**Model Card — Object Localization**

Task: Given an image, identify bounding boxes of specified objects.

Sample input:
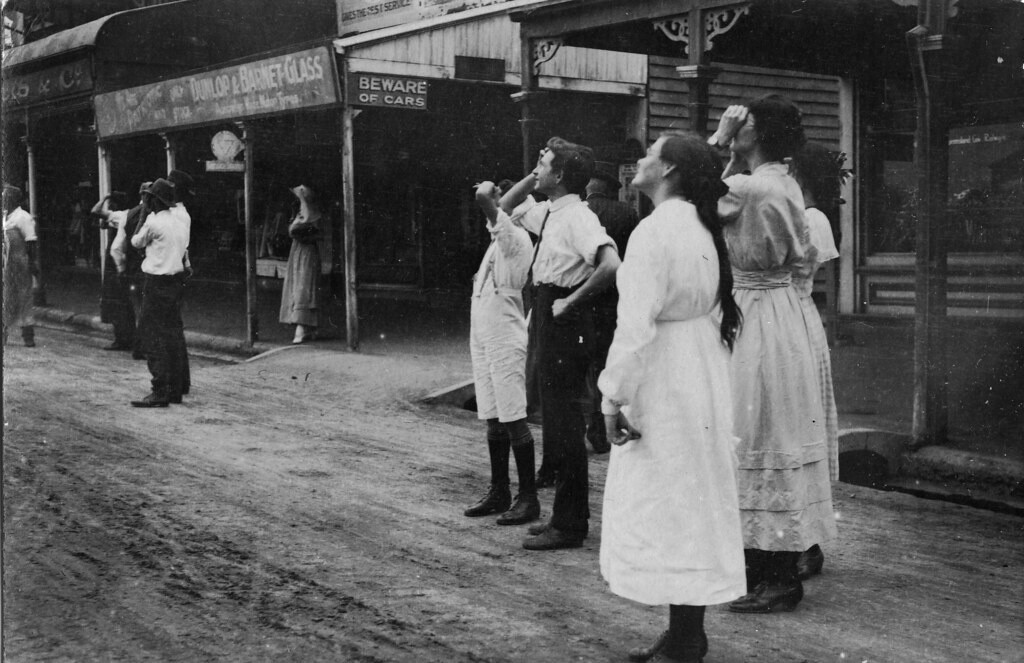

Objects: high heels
[292,329,319,343]
[728,563,802,613]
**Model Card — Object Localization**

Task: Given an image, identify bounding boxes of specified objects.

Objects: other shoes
[22,326,36,347]
[522,517,588,551]
[535,464,559,489]
[629,629,708,663]
[587,412,611,454]
[104,342,130,351]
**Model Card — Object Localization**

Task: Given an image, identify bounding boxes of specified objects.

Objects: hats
[140,178,177,208]
[290,184,315,205]
[167,170,196,196]
[591,161,622,189]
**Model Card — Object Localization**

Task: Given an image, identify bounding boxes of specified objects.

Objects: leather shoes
[168,390,182,403]
[496,489,540,525]
[796,544,824,581]
[464,480,512,516]
[131,392,170,407]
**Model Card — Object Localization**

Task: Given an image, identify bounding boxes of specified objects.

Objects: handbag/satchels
[290,223,324,245]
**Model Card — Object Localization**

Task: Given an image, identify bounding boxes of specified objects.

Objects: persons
[279,181,322,343]
[131,169,194,406]
[597,127,741,663]
[92,182,155,360]
[3,186,37,345]
[463,178,541,522]
[584,92,849,611]
[498,138,621,550]
